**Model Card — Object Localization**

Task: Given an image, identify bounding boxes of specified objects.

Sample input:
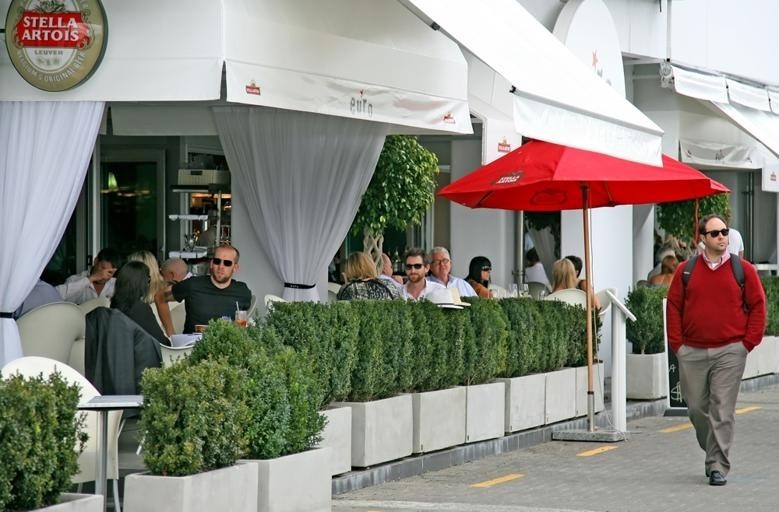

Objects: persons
[646,223,746,289]
[12,244,252,347]
[664,214,768,486]
[329,232,601,311]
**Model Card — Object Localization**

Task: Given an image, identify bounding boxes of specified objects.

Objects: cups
[493,283,547,300]
[235,311,247,328]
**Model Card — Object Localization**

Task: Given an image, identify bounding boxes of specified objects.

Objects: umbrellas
[436,140,733,431]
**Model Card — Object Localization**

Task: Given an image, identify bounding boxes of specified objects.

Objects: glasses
[482,266,492,271]
[406,264,421,269]
[704,229,729,237]
[213,258,232,266]
[433,259,449,264]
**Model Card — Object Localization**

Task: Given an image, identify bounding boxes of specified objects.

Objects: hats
[429,288,464,310]
[450,288,472,306]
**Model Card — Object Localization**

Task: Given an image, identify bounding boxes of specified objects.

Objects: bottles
[386,248,408,273]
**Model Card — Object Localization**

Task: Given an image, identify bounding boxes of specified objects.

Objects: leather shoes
[710,471,726,484]
